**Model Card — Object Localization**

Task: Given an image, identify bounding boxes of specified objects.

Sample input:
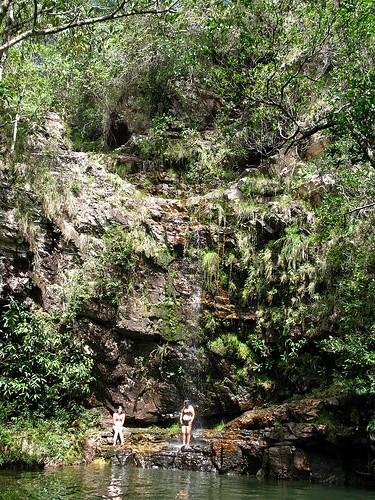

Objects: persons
[112,405,125,446]
[179,400,195,445]
